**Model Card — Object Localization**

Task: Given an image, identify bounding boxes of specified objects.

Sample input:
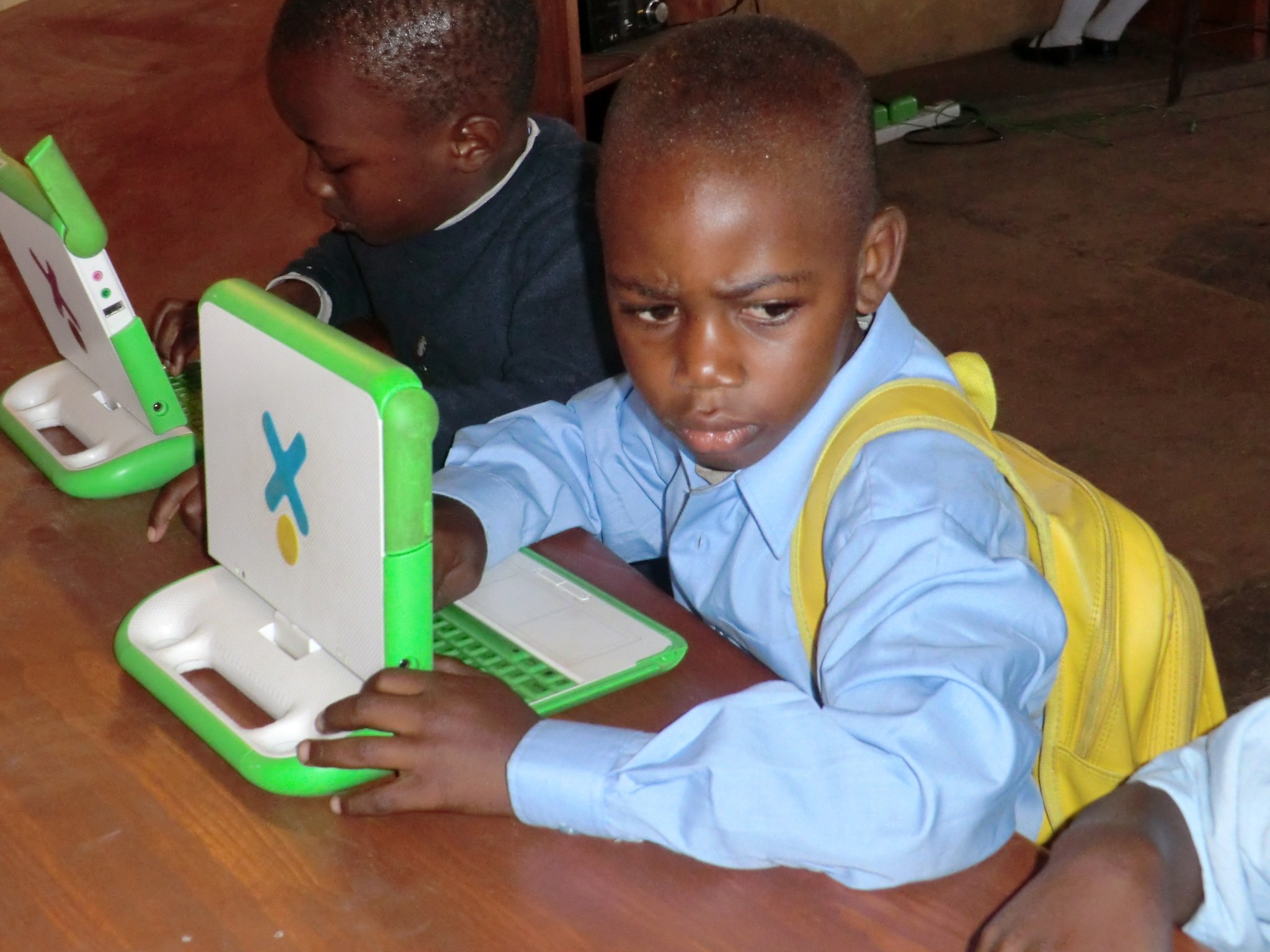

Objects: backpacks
[793,351,1230,852]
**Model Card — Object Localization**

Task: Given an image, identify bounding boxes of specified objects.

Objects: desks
[0,0,1210,952]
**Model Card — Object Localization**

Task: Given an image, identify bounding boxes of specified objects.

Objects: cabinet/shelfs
[567,0,713,150]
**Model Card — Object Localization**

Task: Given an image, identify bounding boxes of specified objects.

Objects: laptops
[0,133,203,499]
[113,277,689,795]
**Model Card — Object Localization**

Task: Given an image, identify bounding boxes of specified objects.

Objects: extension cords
[873,100,963,146]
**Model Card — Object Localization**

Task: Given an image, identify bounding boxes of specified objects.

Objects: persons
[146,0,630,543]
[301,12,1068,891]
[974,694,1270,952]
[1008,0,1147,65]
